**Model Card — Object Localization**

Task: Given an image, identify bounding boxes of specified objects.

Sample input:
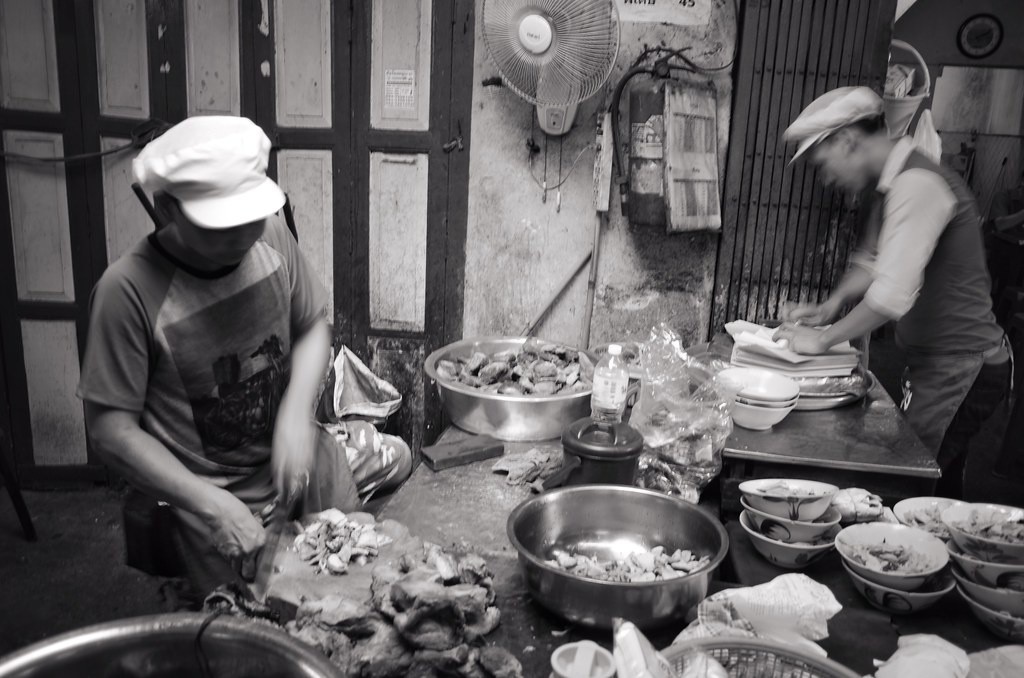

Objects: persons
[75,116,362,601]
[771,87,1015,500]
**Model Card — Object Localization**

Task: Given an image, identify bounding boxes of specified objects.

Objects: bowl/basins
[0,610,350,678]
[423,336,594,442]
[738,478,1024,642]
[507,482,729,628]
[715,367,800,430]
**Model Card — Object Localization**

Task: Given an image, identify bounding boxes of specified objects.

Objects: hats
[131,115,286,230]
[783,86,885,168]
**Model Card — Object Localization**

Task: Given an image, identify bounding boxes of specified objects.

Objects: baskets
[659,636,862,678]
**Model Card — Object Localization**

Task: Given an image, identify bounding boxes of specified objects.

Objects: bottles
[589,344,630,425]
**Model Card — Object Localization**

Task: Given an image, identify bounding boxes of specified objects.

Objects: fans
[479,0,622,135]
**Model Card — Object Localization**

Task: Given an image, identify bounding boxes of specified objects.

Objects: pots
[540,418,644,490]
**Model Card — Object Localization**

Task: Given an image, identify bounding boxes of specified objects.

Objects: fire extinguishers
[611,46,695,228]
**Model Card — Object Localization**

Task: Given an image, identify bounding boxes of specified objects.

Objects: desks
[724,368,938,506]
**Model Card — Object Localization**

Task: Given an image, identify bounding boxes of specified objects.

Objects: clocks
[956,14,1002,58]
